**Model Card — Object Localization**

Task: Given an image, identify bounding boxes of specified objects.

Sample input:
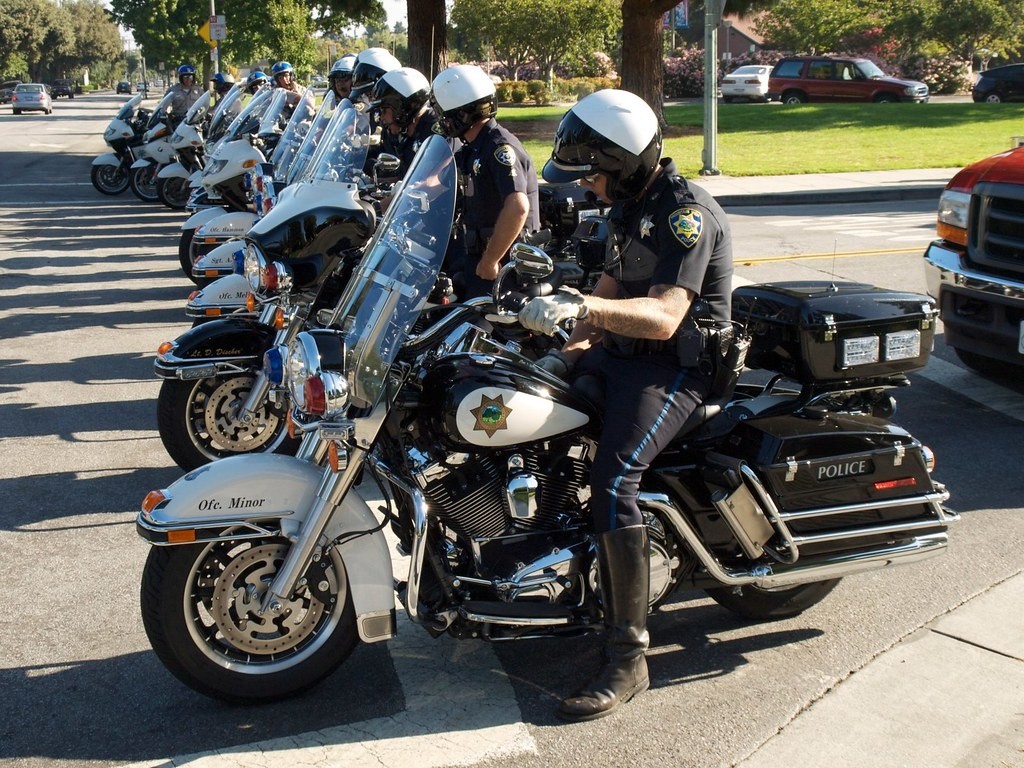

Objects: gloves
[534,348,573,378]
[518,285,589,338]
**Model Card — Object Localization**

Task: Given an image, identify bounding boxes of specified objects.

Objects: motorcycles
[87,77,961,703]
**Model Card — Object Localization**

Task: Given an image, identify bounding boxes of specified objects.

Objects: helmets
[430,64,498,137]
[178,64,196,84]
[542,89,663,201]
[328,48,430,129]
[272,61,292,79]
[245,71,268,91]
[209,72,234,94]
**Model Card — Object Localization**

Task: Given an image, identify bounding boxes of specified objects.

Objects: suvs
[768,56,930,104]
[0,80,23,104]
[51,79,74,99]
[921,142,1024,367]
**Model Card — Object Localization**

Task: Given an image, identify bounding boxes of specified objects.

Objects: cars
[156,80,163,87]
[137,82,150,92]
[972,63,1024,103]
[117,82,131,95]
[12,84,53,115]
[721,65,775,103]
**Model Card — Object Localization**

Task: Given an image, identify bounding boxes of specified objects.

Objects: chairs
[842,66,852,81]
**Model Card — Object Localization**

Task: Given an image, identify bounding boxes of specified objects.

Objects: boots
[553,524,650,722]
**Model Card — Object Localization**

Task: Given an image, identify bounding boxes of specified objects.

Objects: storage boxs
[571,216,609,273]
[729,281,943,382]
[537,182,612,228]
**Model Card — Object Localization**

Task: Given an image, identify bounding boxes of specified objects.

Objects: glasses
[331,74,352,82]
[584,173,600,188]
[181,74,194,79]
[373,108,386,119]
[248,80,265,90]
[277,74,290,78]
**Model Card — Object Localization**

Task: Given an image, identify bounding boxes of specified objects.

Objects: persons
[160,47,538,303]
[524,88,733,721]
